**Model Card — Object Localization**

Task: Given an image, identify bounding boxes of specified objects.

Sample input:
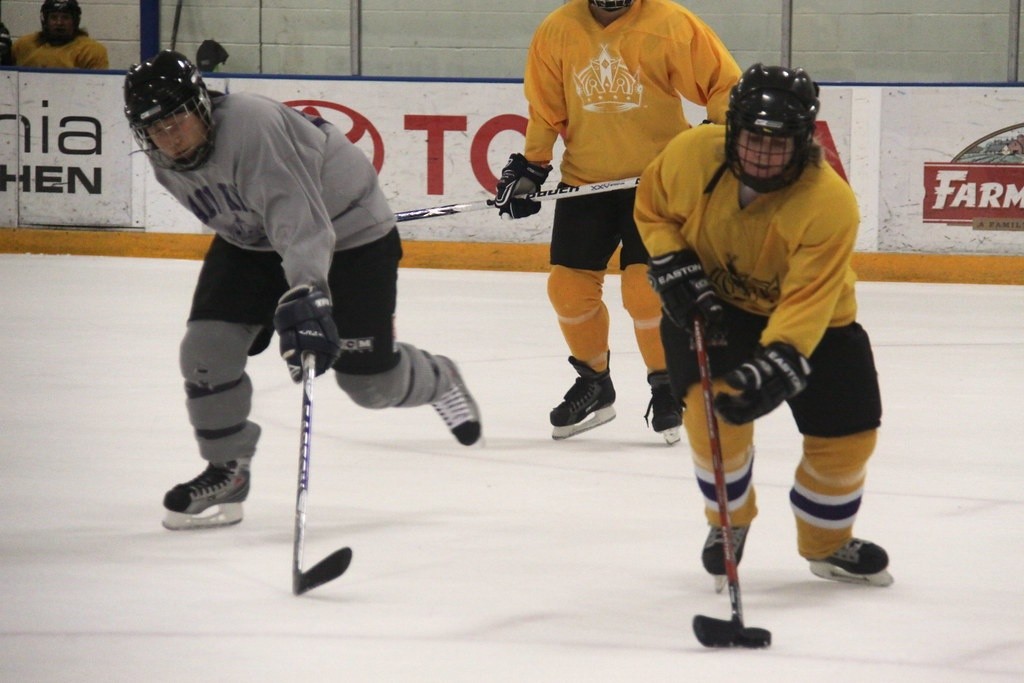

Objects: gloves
[646,248,727,341]
[495,153,553,221]
[713,341,816,424]
[273,285,340,384]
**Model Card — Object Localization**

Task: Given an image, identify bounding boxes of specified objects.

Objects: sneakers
[809,538,893,587]
[432,355,487,451]
[550,350,617,440]
[162,451,251,530]
[702,525,752,594]
[645,369,690,444]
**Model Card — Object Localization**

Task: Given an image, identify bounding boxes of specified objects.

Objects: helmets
[587,0,632,12]
[40,0,81,44]
[724,62,820,193]
[123,50,216,173]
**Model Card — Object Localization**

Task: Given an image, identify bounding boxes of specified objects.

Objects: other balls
[736,628,772,648]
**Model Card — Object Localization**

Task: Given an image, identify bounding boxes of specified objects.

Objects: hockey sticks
[691,308,744,649]
[291,351,353,595]
[394,176,640,222]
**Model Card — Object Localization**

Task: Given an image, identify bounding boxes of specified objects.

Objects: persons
[496,0,742,445]
[12,0,110,70]
[123,50,483,532]
[633,63,895,593]
